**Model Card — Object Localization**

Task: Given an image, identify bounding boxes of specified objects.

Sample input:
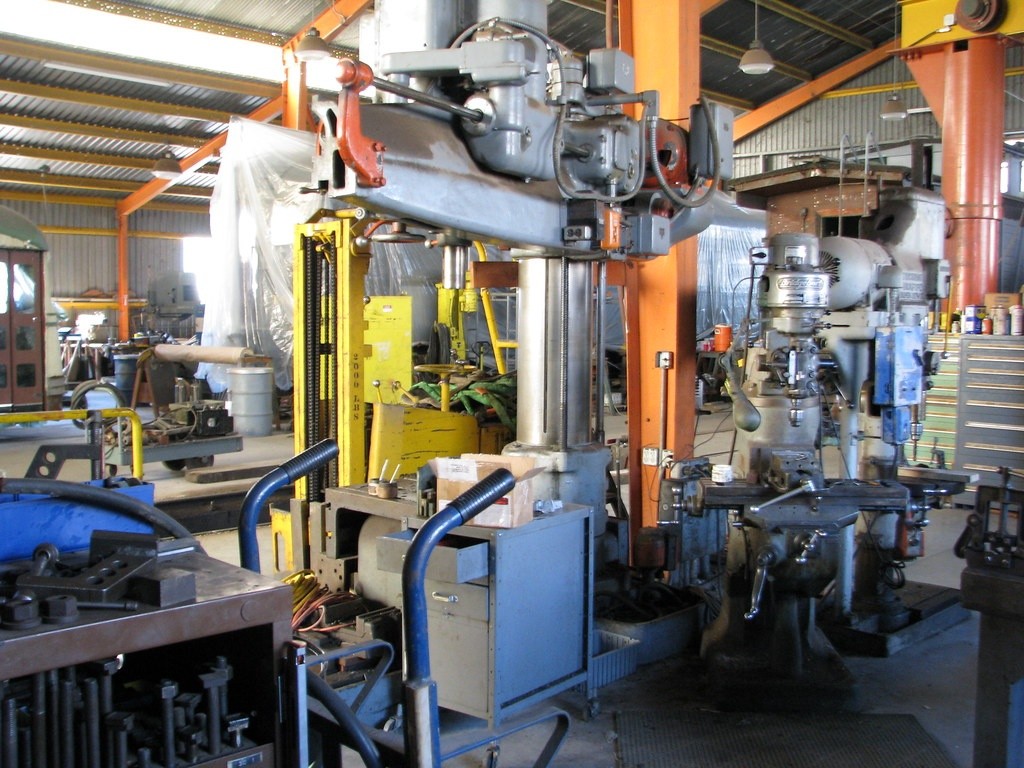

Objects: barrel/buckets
[226,366,274,436]
[112,355,141,407]
[714,324,732,353]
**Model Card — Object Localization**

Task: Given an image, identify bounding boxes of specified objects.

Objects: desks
[695,350,725,403]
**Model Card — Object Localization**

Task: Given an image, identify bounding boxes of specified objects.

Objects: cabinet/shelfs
[375,502,597,726]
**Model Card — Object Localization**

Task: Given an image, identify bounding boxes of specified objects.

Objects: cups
[711,464,732,482]
[368,478,397,498]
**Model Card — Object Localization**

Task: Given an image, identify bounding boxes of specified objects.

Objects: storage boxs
[985,293,1022,314]
[427,452,547,529]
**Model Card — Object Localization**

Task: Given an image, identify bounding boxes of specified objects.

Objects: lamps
[878,1,909,123]
[738,0,776,76]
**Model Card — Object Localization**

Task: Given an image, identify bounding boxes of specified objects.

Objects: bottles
[704,340,708,351]
[1011,305,1023,335]
[951,308,961,333]
[710,338,714,351]
